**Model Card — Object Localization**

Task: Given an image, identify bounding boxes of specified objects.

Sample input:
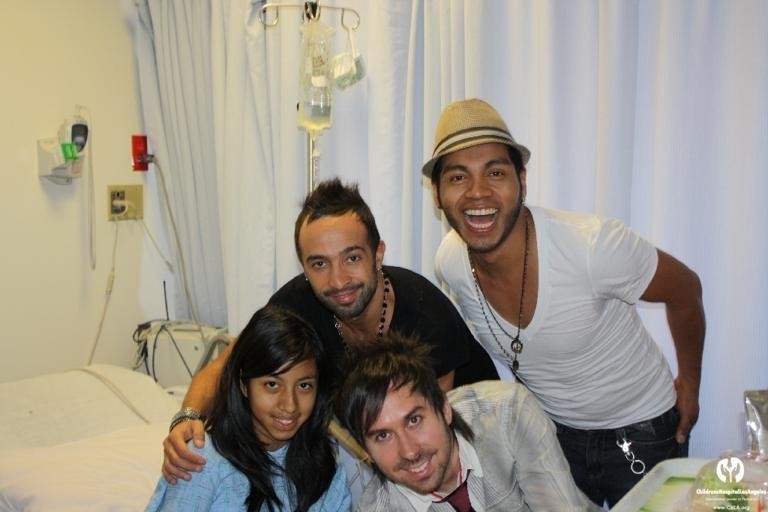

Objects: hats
[418,96,535,179]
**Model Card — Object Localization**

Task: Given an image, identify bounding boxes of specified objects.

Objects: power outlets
[130,135,149,171]
[106,184,144,222]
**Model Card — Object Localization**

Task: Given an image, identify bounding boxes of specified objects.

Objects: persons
[133,305,366,512]
[418,97,706,512]
[160,174,506,484]
[317,322,596,512]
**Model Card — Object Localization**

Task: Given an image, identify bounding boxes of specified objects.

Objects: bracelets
[166,403,205,437]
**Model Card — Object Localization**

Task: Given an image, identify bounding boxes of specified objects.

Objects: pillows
[0,422,177,512]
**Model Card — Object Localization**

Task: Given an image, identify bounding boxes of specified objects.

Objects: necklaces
[465,248,524,372]
[465,210,533,353]
[320,271,389,353]
